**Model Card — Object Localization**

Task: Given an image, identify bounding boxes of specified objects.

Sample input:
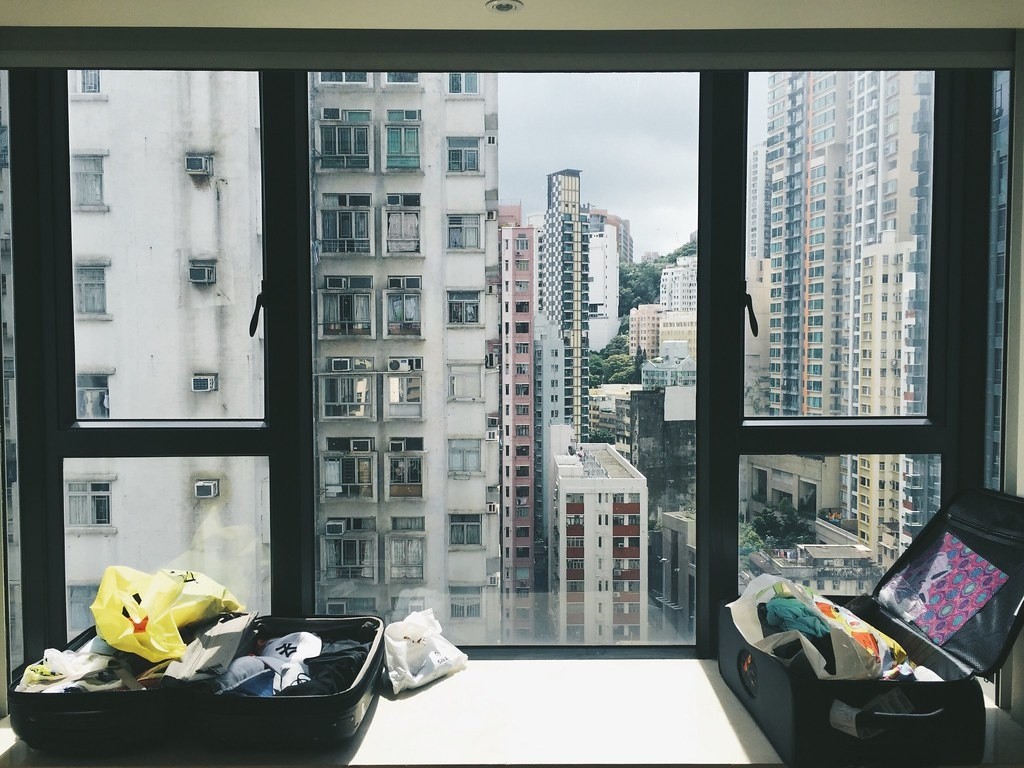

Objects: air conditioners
[890,359,897,365]
[488,416,500,427]
[614,569,621,576]
[519,580,526,585]
[516,497,527,505]
[326,277,348,290]
[194,479,219,499]
[486,573,498,586]
[326,601,346,615]
[350,440,372,453]
[326,520,346,536]
[386,195,402,207]
[191,376,215,392]
[408,601,424,614]
[618,543,625,548]
[387,358,409,372]
[320,107,342,120]
[331,357,352,371]
[488,353,496,367]
[486,502,497,513]
[486,135,496,147]
[613,592,620,598]
[488,284,497,294]
[389,440,405,453]
[188,267,213,282]
[404,109,420,122]
[485,428,497,441]
[405,277,422,289]
[185,156,209,173]
[486,211,496,221]
